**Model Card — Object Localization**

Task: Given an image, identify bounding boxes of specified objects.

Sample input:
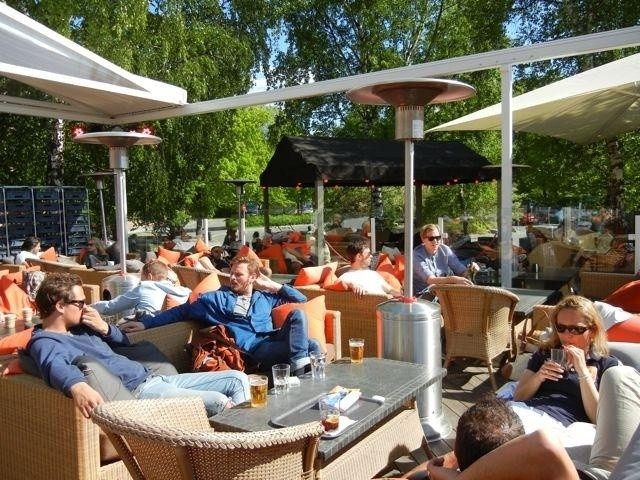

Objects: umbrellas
[424,52,640,145]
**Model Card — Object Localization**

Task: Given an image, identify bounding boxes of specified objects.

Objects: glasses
[425,236,441,241]
[556,322,594,335]
[63,299,84,310]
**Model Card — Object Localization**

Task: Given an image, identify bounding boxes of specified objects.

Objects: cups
[22,306,33,323]
[348,337,365,364]
[309,350,327,380]
[272,363,290,395]
[319,395,340,429]
[550,348,569,379]
[249,375,269,408]
[4,314,16,329]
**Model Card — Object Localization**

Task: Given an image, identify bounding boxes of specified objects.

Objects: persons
[14,208,640,480]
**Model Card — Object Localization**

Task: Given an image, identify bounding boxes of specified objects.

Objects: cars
[246,201,260,213]
[516,208,601,225]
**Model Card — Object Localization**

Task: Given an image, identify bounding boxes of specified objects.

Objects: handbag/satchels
[191,324,246,372]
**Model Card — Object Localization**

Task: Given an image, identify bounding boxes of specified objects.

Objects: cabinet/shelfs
[7,187,91,253]
